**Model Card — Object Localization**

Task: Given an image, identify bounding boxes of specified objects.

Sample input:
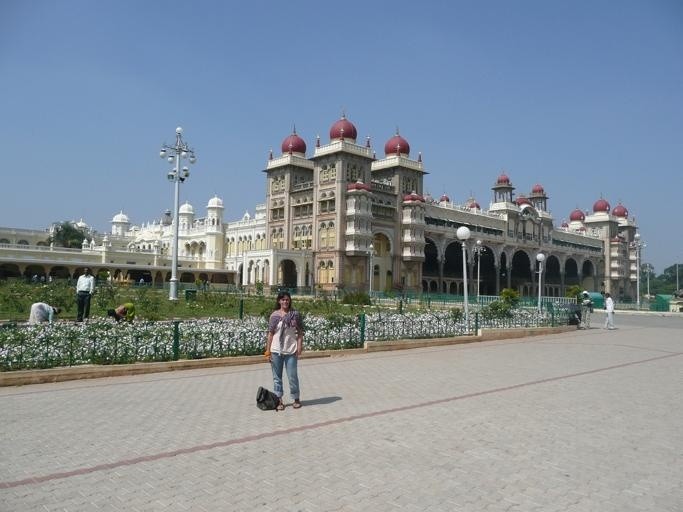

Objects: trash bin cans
[186,291,197,305]
[583,292,604,308]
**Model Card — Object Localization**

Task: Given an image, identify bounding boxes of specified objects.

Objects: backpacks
[257,386,279,410]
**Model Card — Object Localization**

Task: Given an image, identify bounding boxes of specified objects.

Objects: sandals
[293,402,301,408]
[276,403,284,410]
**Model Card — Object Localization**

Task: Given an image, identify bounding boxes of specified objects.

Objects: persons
[73,268,97,325]
[139,277,144,287]
[30,273,53,285]
[576,290,594,330]
[262,291,304,411]
[602,292,617,329]
[108,302,135,324]
[27,300,61,323]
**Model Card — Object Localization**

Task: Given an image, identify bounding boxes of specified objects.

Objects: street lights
[537,253,545,314]
[456,226,470,335]
[631,232,651,305]
[159,127,196,300]
[365,244,377,299]
